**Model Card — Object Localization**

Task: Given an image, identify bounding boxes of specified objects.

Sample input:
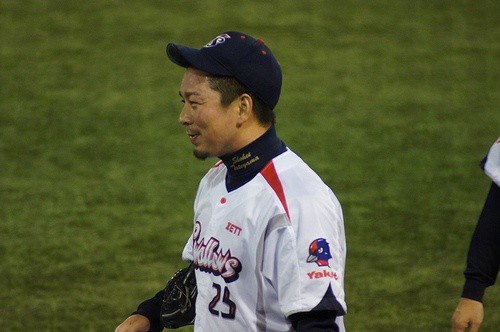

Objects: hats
[166,31,282,111]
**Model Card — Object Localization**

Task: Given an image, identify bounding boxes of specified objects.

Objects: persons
[450,137,500,332]
[114,32,348,332]
